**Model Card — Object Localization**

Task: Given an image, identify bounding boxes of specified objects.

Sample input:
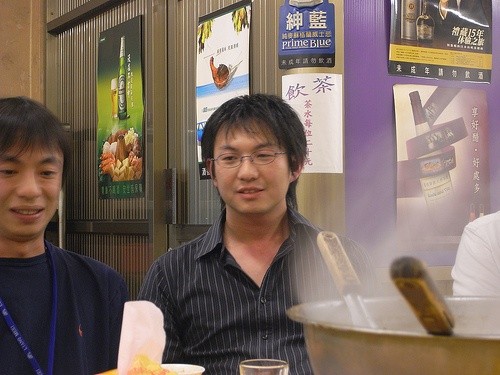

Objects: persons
[136,93,381,375]
[450,211,500,297]
[0,96,132,375]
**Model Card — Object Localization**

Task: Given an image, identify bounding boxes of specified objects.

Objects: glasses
[209,149,288,168]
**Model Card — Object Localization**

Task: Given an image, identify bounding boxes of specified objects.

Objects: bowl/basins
[287,296,500,375]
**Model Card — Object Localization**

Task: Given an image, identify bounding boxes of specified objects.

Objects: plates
[162,364,205,375]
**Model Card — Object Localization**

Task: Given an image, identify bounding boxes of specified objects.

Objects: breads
[127,352,181,375]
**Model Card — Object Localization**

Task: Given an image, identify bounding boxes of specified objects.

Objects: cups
[111,78,118,119]
[239,359,289,375]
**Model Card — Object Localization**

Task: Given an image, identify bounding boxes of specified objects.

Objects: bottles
[415,0,435,43]
[118,36,128,120]
[410,91,455,216]
